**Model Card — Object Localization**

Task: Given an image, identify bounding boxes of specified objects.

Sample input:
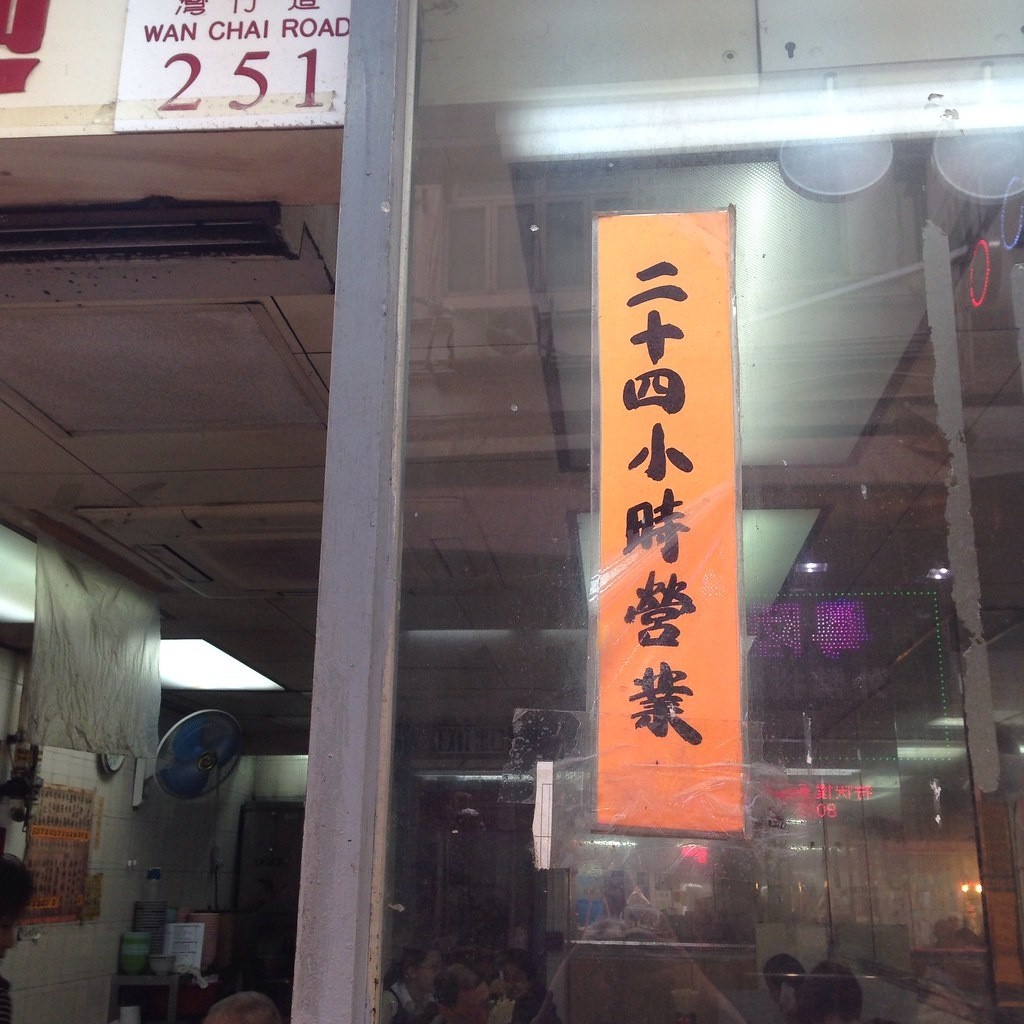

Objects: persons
[378,884,1003,1023]
[1,852,36,1024]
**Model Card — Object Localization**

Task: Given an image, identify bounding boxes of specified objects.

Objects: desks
[106,961,253,1024]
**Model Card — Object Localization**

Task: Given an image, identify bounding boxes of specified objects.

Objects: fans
[131,708,246,809]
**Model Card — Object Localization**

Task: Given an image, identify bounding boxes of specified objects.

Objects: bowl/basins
[133,899,168,975]
[149,955,175,976]
[176,906,193,923]
[119,931,151,976]
[190,911,219,972]
